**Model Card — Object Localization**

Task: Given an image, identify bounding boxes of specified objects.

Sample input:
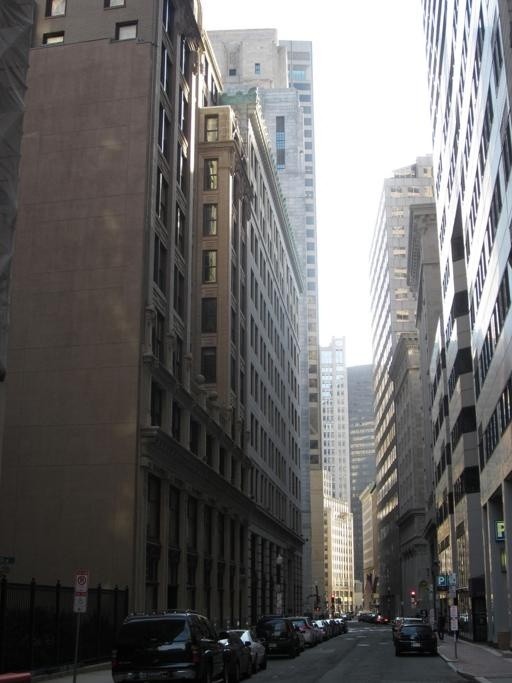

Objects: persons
[437,611,445,640]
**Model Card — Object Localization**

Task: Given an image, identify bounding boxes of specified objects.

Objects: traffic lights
[414,600,420,606]
[317,595,321,604]
[411,592,416,598]
[331,594,335,603]
[316,606,321,611]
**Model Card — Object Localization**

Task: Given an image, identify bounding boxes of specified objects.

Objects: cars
[392,615,439,659]
[357,611,390,624]
[108,608,354,682]
